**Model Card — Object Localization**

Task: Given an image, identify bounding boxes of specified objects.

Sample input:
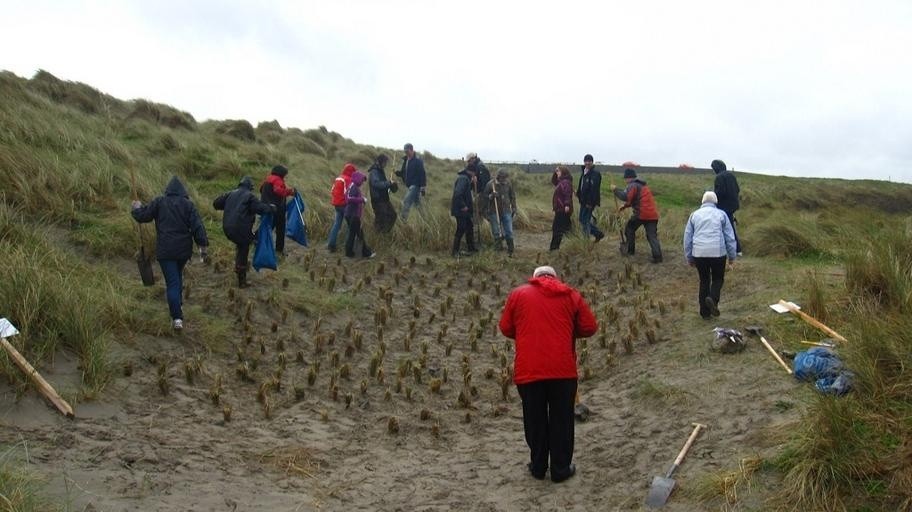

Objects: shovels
[473,175,482,251]
[768,299,849,345]
[744,324,794,376]
[609,171,629,257]
[128,169,156,287]
[644,422,708,511]
[0,316,75,421]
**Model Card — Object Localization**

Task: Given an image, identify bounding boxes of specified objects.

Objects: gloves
[510,208,517,218]
[362,196,368,208]
[131,199,142,210]
[420,187,426,197]
[288,187,298,197]
[389,181,398,194]
[197,245,211,262]
[489,192,500,200]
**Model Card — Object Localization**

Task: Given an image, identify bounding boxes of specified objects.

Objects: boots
[237,270,252,289]
[495,238,503,251]
[506,238,515,258]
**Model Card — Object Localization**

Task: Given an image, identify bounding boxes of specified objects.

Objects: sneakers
[451,246,479,257]
[345,250,356,258]
[550,463,576,484]
[622,247,636,256]
[328,244,337,252]
[362,252,377,260]
[647,255,663,263]
[528,461,548,481]
[698,294,720,321]
[736,250,743,257]
[172,318,184,330]
[594,231,605,243]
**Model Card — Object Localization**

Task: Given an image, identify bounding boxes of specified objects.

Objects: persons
[259,165,298,257]
[499,264,598,481]
[367,154,397,232]
[450,151,668,265]
[326,162,356,253]
[710,160,744,257]
[395,143,430,225]
[345,171,379,258]
[131,175,211,330]
[214,174,276,288]
[684,191,736,320]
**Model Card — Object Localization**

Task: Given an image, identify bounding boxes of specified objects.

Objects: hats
[583,154,594,162]
[533,266,557,279]
[623,168,638,178]
[465,152,476,162]
